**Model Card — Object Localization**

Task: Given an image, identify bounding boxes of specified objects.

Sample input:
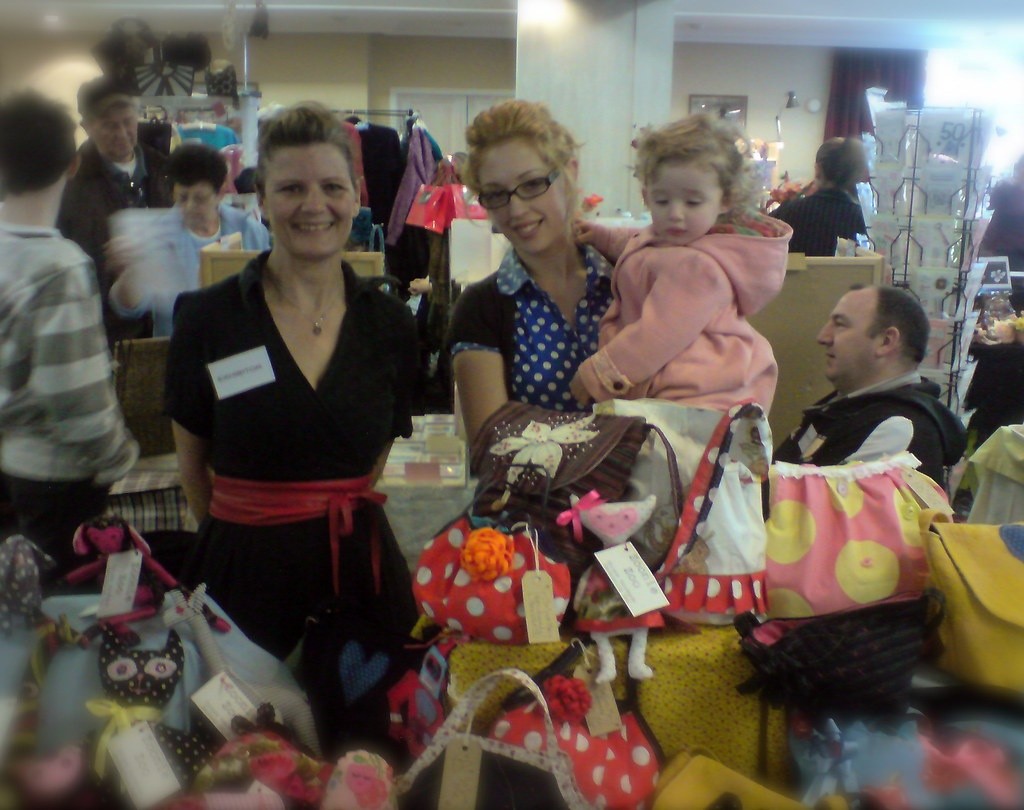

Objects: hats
[78,76,138,123]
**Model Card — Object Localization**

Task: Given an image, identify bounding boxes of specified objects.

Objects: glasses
[478,158,570,209]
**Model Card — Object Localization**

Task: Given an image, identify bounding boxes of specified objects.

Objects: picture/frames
[689,93,750,130]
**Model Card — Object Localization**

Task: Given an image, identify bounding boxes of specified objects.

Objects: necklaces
[263,268,343,337]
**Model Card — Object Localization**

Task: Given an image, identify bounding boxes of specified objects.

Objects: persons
[0,75,1024,775]
[567,112,795,417]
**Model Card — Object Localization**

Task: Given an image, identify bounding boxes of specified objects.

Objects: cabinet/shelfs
[858,104,987,483]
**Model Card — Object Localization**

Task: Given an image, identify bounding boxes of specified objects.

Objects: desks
[112,437,478,566]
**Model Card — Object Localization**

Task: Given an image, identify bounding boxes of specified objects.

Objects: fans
[91,15,160,82]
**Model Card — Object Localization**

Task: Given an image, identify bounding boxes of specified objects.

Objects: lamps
[776,90,801,143]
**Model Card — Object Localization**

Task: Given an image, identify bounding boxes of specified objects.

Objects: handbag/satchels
[137,116,172,154]
[444,160,489,229]
[390,419,1024,810]
[404,161,449,234]
